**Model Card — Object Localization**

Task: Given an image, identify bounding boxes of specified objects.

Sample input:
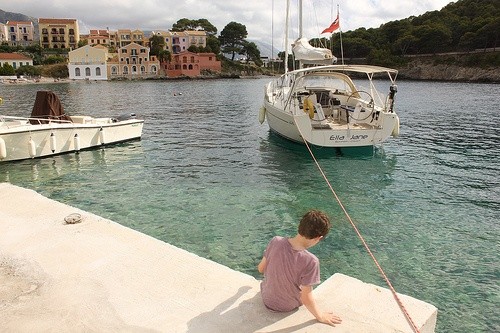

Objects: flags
[322,13,340,34]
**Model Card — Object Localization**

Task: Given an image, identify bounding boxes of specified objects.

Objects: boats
[0,91,145,162]
[259,1,400,148]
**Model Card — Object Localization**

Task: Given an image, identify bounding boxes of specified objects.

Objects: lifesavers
[303,98,314,119]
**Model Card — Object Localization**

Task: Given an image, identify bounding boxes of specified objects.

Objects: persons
[256,209,344,327]
